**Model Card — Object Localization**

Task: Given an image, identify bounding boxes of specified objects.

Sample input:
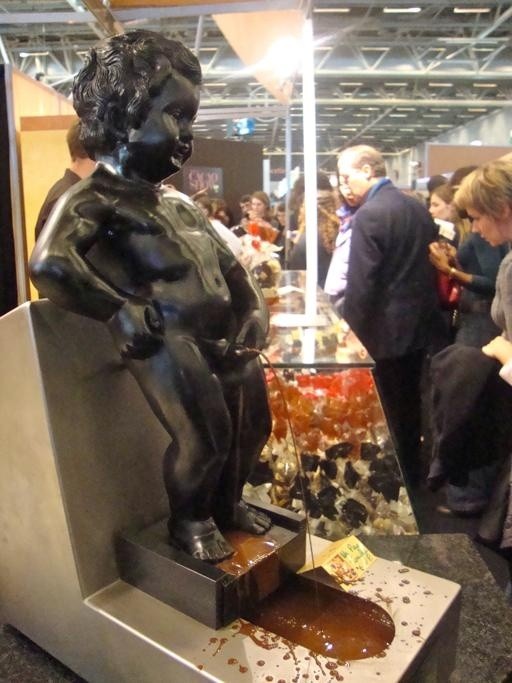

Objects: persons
[28,29,272,563]
[35,118,97,241]
